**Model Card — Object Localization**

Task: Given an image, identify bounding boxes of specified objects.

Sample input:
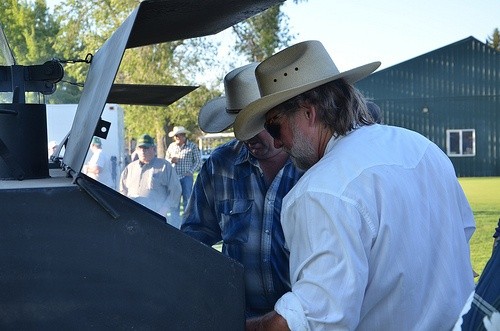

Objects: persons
[451,217,500,331]
[118,132,182,230]
[47,141,60,159]
[81,135,106,181]
[233,40,477,331]
[163,125,202,212]
[179,60,308,319]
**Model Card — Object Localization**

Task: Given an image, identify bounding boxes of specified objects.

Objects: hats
[198,61,261,134]
[137,134,154,149]
[92,136,101,146]
[168,126,191,137]
[234,41,381,142]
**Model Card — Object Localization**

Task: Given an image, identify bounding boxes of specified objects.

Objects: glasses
[264,104,297,140]
